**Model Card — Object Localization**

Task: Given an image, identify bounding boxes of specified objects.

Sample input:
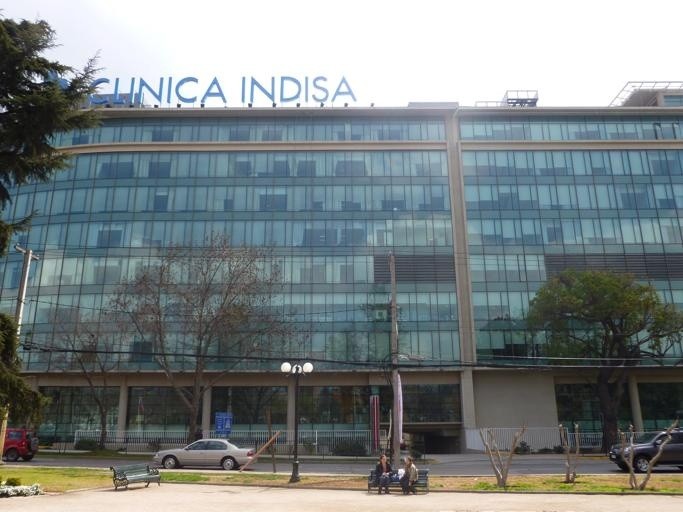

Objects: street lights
[281,361,314,483]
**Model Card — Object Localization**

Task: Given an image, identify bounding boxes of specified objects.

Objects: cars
[153,438,258,470]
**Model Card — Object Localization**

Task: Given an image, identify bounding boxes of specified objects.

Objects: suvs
[608,426,683,473]
[3,427,40,462]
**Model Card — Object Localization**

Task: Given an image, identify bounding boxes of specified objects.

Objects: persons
[399,455,418,495]
[375,453,393,495]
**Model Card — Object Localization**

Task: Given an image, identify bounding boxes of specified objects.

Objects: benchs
[366,469,430,495]
[109,463,161,492]
